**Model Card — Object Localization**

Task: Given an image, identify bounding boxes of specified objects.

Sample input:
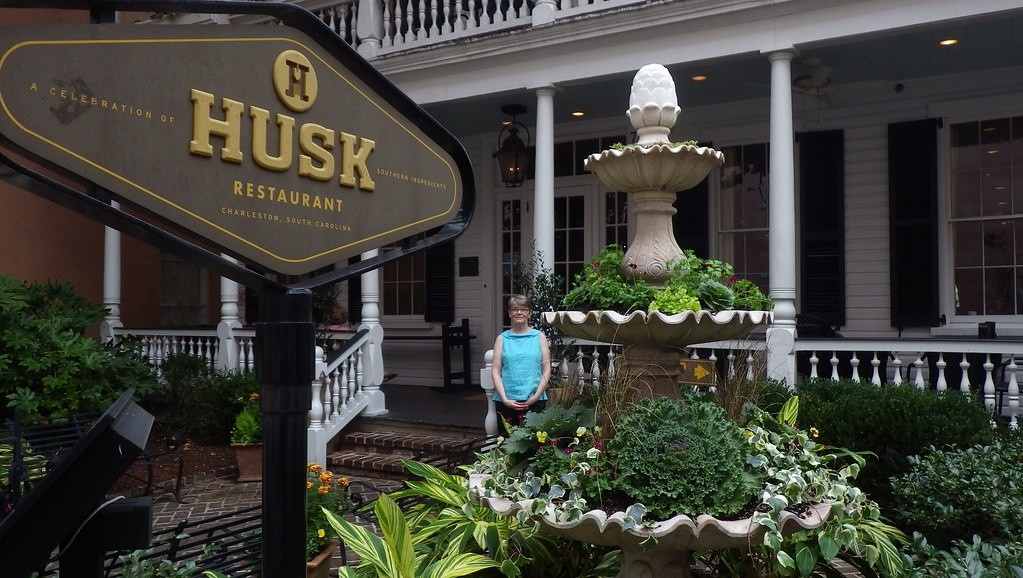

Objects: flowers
[557,242,774,316]
[470,389,879,522]
[305,462,353,564]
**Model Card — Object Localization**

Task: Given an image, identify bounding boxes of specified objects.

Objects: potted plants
[582,138,726,282]
[228,400,266,482]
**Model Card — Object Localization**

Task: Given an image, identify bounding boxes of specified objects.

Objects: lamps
[0,385,156,578]
[491,103,530,188]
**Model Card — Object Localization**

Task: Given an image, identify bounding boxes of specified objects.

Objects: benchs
[0,405,183,507]
[37,504,263,578]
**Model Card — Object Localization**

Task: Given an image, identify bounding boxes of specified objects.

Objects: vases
[456,473,858,578]
[540,307,774,404]
[305,539,340,578]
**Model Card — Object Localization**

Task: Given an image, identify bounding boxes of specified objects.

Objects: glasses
[509,308,530,315]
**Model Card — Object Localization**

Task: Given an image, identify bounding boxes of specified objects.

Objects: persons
[491,294,551,438]
